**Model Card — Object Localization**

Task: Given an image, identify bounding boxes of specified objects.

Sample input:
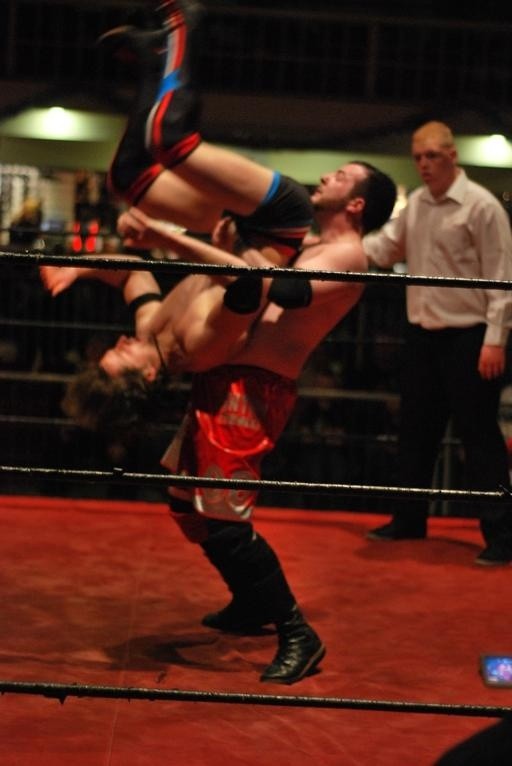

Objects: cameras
[480,654,512,687]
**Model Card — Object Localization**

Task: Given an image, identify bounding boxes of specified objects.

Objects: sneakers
[368,521,426,540]
[473,546,511,565]
[98,23,167,56]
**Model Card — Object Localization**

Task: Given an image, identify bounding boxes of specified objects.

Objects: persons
[362,120,512,564]
[156,157,396,685]
[1,197,512,503]
[62,0,309,405]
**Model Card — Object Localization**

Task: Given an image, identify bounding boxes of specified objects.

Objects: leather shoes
[201,597,263,636]
[257,624,326,682]
